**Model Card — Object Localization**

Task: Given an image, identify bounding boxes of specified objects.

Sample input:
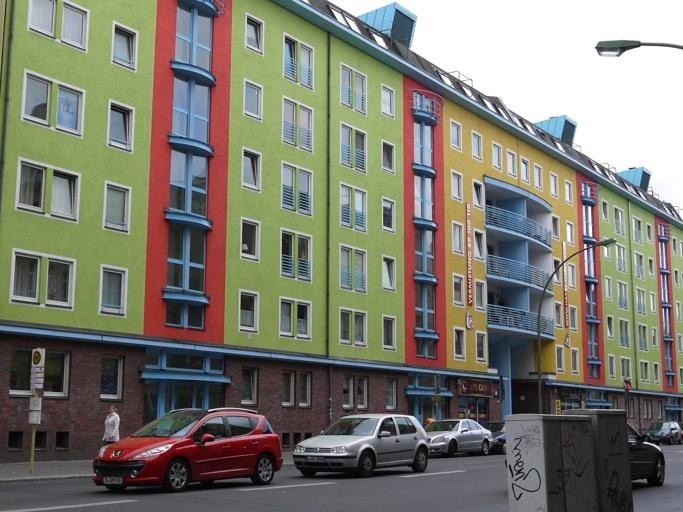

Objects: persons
[95,404,122,477]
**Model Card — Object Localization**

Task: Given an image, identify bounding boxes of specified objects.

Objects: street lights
[595,39,683,59]
[535,238,617,414]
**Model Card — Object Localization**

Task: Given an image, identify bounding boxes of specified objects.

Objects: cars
[641,419,683,445]
[424,418,507,458]
[93,407,283,492]
[292,412,431,478]
[626,421,665,486]
